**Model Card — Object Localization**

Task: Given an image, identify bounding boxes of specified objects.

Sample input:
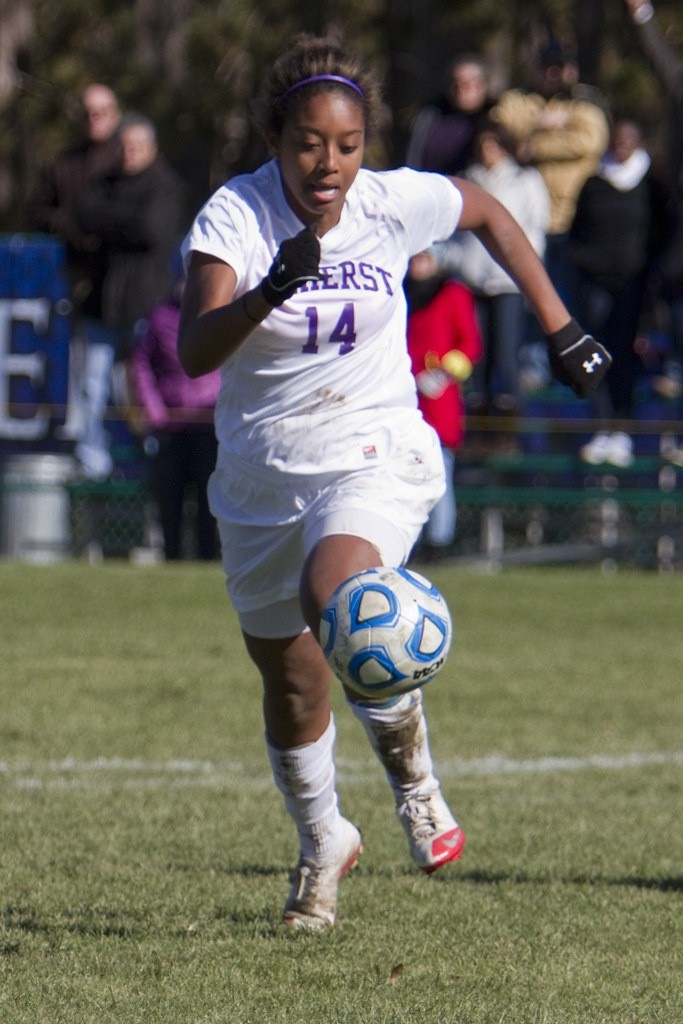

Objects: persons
[399,242,479,558]
[383,54,681,574]
[172,45,611,929]
[128,272,222,563]
[21,71,182,476]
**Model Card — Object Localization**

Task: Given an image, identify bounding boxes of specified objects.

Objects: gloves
[260,222,324,306]
[545,317,613,400]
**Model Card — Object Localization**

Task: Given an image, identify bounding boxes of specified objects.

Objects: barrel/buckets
[0,455,75,562]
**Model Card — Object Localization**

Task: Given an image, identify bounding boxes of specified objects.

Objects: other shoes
[417,545,450,568]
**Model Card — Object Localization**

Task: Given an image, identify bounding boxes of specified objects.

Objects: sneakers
[283,820,364,934]
[395,783,464,874]
